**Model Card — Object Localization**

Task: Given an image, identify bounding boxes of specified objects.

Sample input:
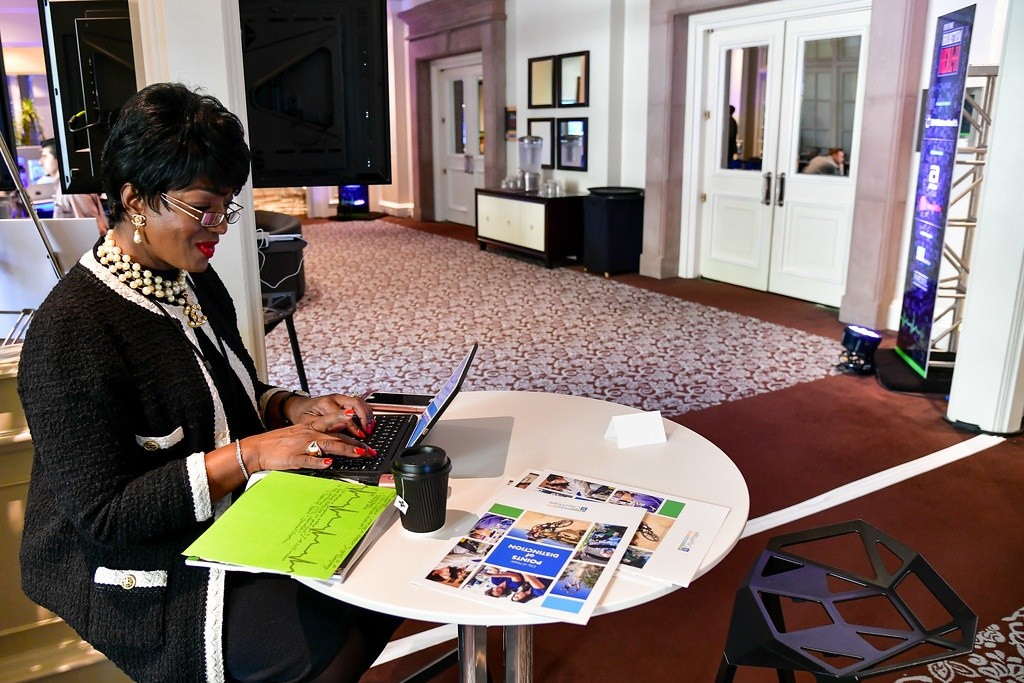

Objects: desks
[245,390,749,683]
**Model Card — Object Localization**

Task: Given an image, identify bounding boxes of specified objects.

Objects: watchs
[278,390,311,423]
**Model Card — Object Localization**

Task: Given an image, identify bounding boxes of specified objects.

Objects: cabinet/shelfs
[474,188,590,268]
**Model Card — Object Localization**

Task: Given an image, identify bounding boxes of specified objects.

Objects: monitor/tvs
[37,0,393,194]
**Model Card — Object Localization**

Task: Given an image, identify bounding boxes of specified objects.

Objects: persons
[469,513,506,538]
[560,578,581,595]
[802,148,844,176]
[485,570,553,603]
[547,475,611,498]
[17,83,403,683]
[728,106,738,167]
[526,525,582,542]
[426,546,483,582]
[597,530,622,558]
[35,138,59,185]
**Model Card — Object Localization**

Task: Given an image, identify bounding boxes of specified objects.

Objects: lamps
[840,324,882,376]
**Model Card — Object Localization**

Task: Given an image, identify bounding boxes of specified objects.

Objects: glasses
[159,192,243,227]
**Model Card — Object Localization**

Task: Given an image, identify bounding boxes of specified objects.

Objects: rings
[345,392,357,397]
[306,441,322,457]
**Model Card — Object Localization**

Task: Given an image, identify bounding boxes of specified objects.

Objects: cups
[537,179,564,198]
[389,445,452,533]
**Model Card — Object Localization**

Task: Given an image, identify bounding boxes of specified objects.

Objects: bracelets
[236,439,249,481]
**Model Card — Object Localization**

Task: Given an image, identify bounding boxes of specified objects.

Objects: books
[185,477,382,579]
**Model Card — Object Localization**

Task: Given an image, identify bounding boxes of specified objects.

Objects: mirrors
[528,55,556,108]
[556,50,589,108]
[557,117,587,171]
[528,118,555,169]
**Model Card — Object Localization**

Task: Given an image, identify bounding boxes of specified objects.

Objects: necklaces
[97,229,207,327]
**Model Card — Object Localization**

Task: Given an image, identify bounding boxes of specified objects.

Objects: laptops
[281,343,479,484]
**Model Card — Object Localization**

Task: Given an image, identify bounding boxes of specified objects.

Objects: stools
[262,292,310,394]
[715,519,979,683]
